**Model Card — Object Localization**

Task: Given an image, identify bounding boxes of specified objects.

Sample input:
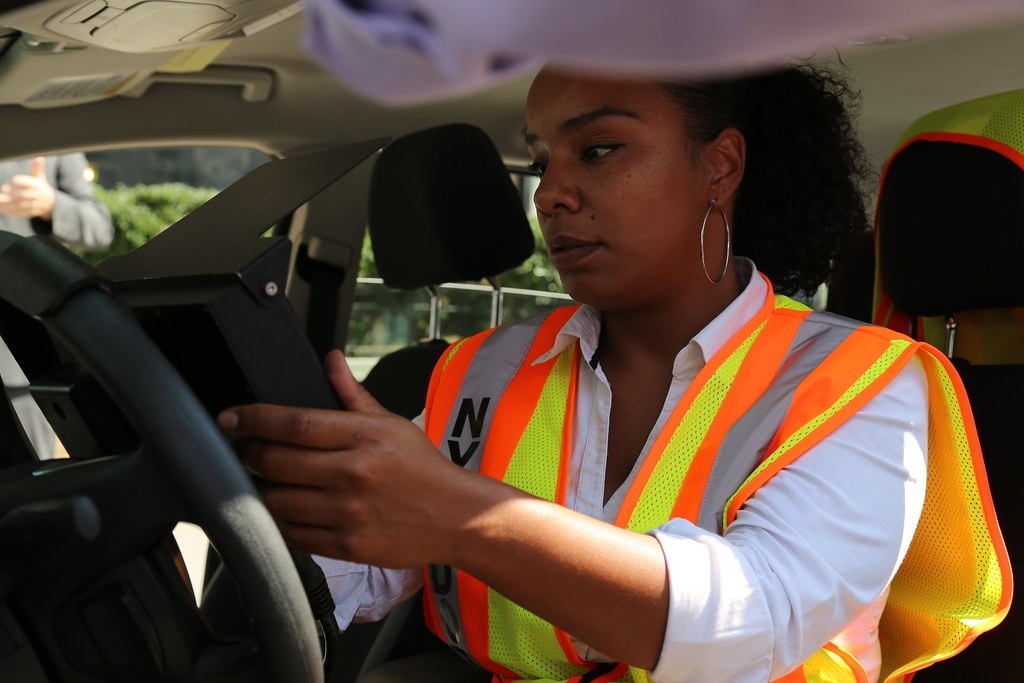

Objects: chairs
[361,123,536,421]
[867,86,1024,683]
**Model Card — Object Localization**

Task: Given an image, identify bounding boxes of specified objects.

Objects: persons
[0,146,114,460]
[217,60,1015,683]
